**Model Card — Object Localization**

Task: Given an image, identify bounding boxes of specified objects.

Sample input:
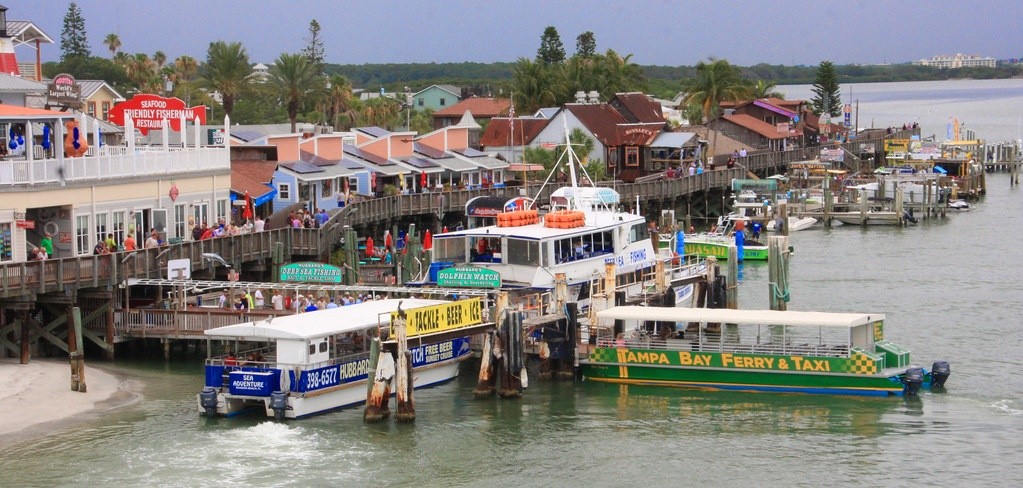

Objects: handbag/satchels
[110,239,118,252]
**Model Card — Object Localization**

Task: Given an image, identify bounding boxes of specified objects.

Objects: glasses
[46,235,51,236]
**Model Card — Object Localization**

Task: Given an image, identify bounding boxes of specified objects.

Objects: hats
[376,295,381,299]
[344,291,350,295]
[233,297,242,304]
[227,351,237,356]
[295,294,305,298]
[358,294,363,298]
[213,223,220,227]
[254,355,263,360]
[240,290,246,295]
[223,288,228,292]
[690,163,698,168]
[298,209,304,214]
[308,294,313,297]
[366,294,373,298]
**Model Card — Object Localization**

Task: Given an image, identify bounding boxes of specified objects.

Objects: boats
[581,303,952,398]
[195,296,496,419]
[793,200,911,226]
[423,107,717,326]
[846,174,943,203]
[651,210,773,260]
[758,130,988,227]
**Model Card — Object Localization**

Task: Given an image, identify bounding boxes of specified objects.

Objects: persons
[191,215,264,240]
[144,228,163,248]
[903,121,916,130]
[337,189,346,207]
[733,147,747,156]
[727,158,733,168]
[124,233,135,251]
[668,166,682,178]
[752,219,762,244]
[347,192,355,203]
[887,126,896,134]
[689,163,703,175]
[94,233,116,255]
[218,289,385,312]
[27,231,53,261]
[286,207,330,228]
